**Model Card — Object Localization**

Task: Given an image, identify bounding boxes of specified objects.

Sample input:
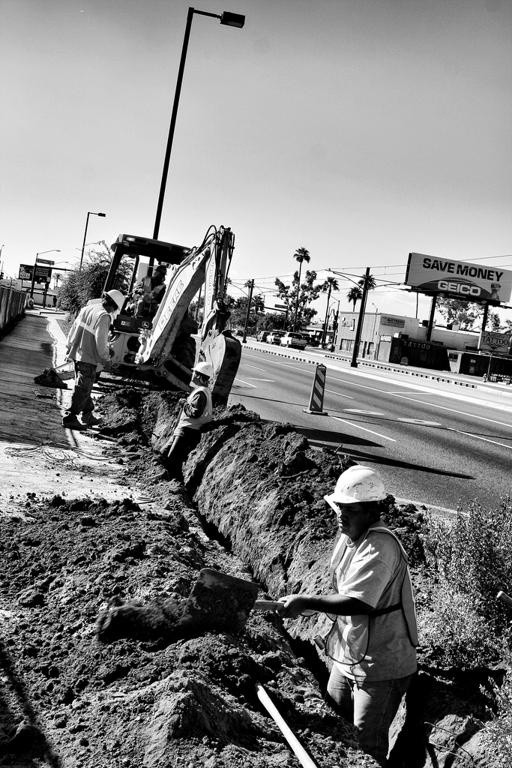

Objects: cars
[235,329,308,350]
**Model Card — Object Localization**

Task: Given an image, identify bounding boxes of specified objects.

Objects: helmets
[326,465,388,505]
[103,289,126,311]
[190,362,214,378]
[153,265,168,276]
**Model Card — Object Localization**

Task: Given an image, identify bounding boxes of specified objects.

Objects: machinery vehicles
[90,223,243,406]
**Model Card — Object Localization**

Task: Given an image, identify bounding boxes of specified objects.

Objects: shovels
[179,569,288,630]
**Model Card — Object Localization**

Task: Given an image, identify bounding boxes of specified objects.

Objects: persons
[142,263,168,311]
[275,463,420,761]
[160,361,215,466]
[60,288,125,431]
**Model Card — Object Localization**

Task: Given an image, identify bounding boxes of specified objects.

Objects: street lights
[79,212,107,272]
[42,261,69,308]
[29,249,61,303]
[146,4,246,279]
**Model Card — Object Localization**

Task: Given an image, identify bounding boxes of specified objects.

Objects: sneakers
[62,419,88,431]
[81,414,102,425]
